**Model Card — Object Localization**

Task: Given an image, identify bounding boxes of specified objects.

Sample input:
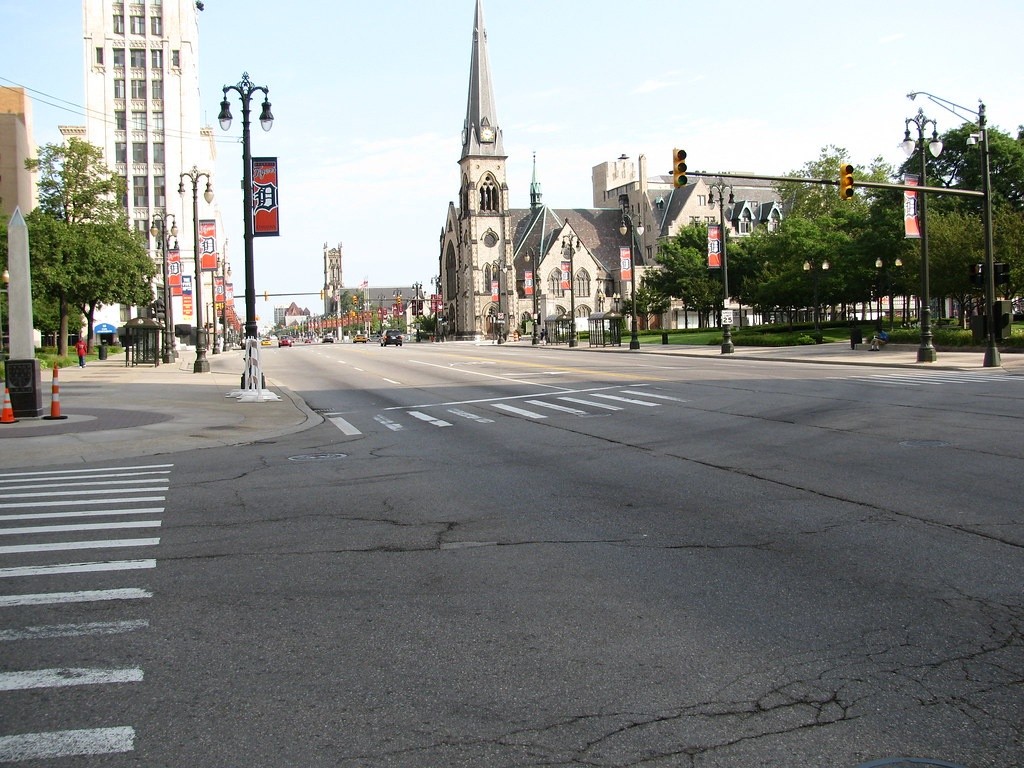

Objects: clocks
[462,129,467,146]
[480,127,495,143]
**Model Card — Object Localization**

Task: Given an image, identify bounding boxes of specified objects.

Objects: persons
[75,337,87,368]
[867,327,889,351]
[540,328,546,346]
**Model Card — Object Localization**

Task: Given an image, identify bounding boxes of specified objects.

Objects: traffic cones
[1,388,18,424]
[41,361,68,422]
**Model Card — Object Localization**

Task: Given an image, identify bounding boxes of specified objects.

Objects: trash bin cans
[662,333,668,344]
[851,328,862,350]
[99,346,107,360]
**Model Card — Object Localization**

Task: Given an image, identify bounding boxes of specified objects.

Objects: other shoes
[875,349,879,351]
[83,365,86,368]
[79,366,82,369]
[868,349,874,351]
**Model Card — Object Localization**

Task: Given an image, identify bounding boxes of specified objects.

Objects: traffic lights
[840,162,854,200]
[673,148,688,187]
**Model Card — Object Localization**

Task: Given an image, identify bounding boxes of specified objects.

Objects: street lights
[619,201,644,350]
[216,70,276,388]
[492,257,508,344]
[177,164,215,372]
[562,230,582,347]
[708,175,737,353]
[150,206,181,363]
[299,276,443,343]
[524,245,544,345]
[902,106,944,363]
[908,89,1001,367]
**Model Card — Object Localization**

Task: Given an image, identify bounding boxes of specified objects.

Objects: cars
[261,340,271,346]
[278,335,293,348]
[380,328,402,347]
[322,335,334,343]
[353,334,367,343]
